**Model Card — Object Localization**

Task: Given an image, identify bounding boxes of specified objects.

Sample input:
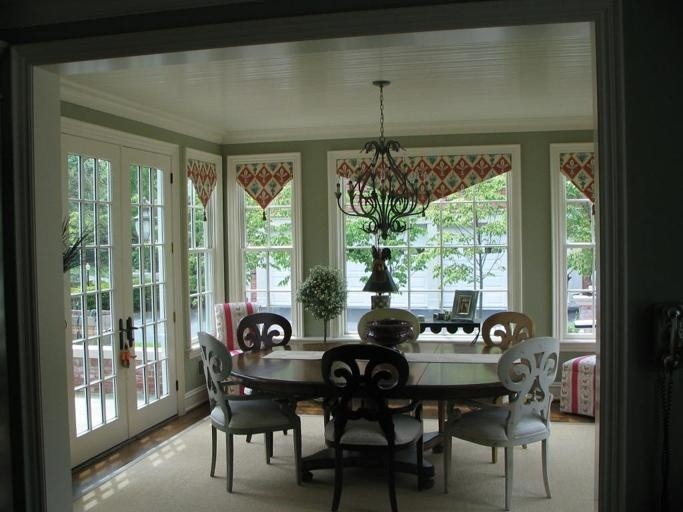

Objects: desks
[230,350,522,489]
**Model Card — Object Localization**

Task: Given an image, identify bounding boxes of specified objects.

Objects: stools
[561,352,598,416]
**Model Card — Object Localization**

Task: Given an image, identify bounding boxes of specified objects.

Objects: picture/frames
[450,291,480,321]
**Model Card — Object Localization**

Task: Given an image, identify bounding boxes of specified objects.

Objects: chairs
[444,334,560,511]
[198,332,304,491]
[236,312,292,442]
[214,302,260,396]
[357,309,420,342]
[480,311,534,348]
[321,344,422,511]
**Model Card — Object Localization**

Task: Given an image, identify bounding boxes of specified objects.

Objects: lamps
[335,80,429,236]
[361,270,398,312]
